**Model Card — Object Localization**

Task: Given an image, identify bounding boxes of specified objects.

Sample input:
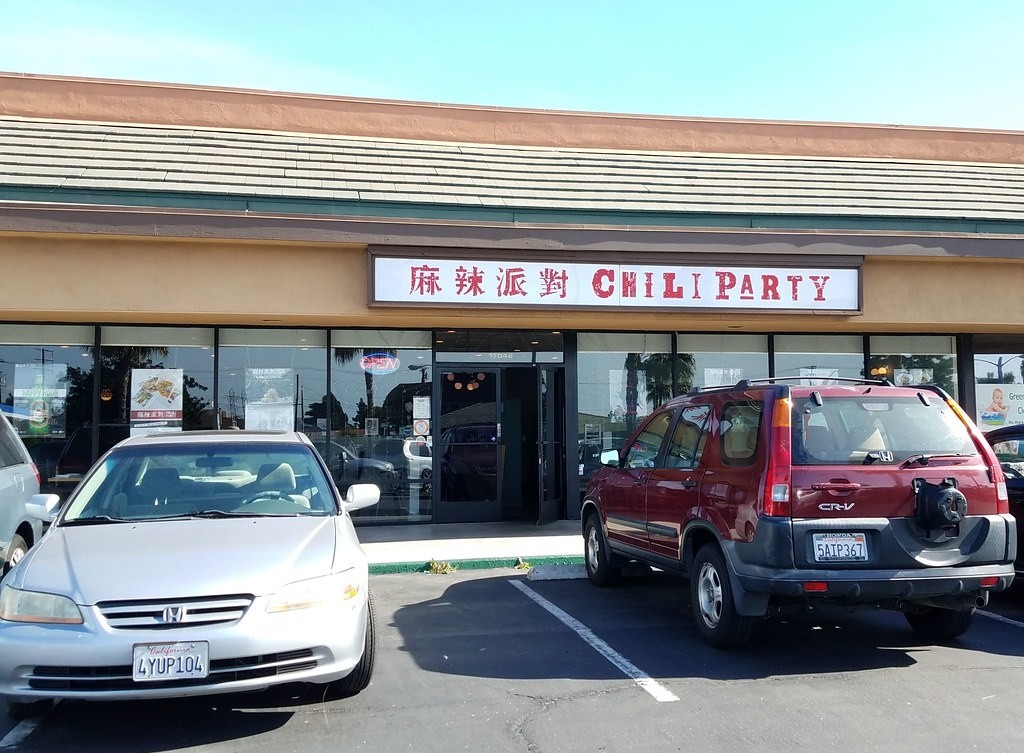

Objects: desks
[48,475,84,481]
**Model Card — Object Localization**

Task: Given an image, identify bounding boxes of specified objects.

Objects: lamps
[99,386,113,401]
[870,367,887,380]
[446,331,485,391]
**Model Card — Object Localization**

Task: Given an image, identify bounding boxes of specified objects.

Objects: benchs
[131,474,319,509]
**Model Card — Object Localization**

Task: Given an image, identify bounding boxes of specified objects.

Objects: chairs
[125,468,198,516]
[844,426,885,462]
[806,426,836,459]
[240,462,311,510]
[739,427,758,458]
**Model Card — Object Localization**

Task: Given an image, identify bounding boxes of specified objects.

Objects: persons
[986,388,1010,415]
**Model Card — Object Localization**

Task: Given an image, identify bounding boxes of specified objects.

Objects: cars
[0,427,381,719]
[308,438,433,503]
[985,424,1024,588]
[578,436,653,492]
[0,407,43,580]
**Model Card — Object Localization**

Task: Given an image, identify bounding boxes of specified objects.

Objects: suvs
[440,421,498,493]
[579,375,1018,650]
